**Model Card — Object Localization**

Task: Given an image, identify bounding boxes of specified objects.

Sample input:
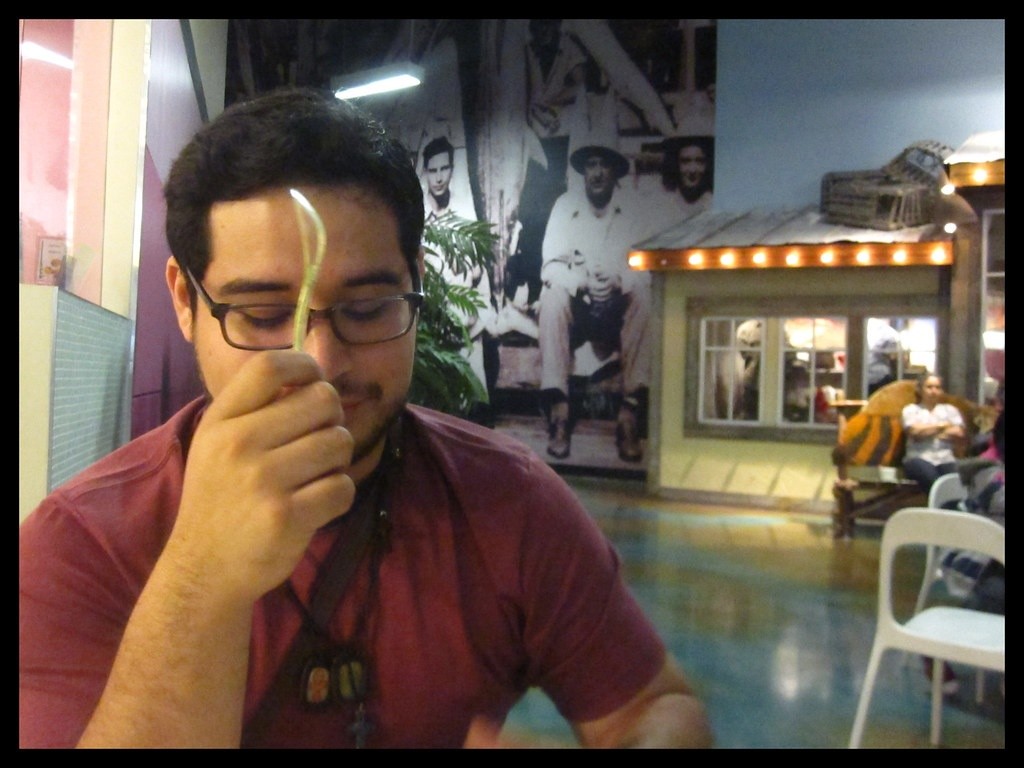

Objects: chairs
[849,470,1006,749]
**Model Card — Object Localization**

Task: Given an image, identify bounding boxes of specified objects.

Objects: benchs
[826,378,1006,540]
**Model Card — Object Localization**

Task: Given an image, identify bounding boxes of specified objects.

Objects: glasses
[175,253,426,350]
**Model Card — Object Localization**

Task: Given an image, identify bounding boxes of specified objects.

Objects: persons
[231,19,713,463]
[900,374,1005,695]
[20,83,709,750]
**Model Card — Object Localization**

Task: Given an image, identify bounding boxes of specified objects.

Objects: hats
[569,134,629,177]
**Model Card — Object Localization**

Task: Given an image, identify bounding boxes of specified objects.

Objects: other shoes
[616,420,643,461]
[547,418,569,457]
